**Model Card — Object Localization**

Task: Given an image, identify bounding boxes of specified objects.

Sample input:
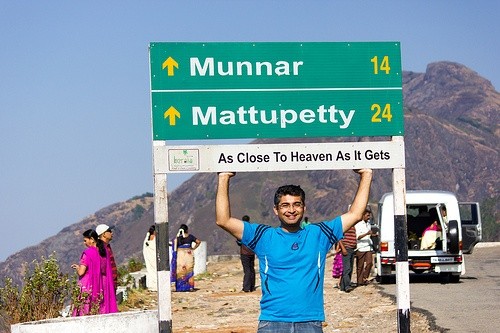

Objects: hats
[96,224,116,238]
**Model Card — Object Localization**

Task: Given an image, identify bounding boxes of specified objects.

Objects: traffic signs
[151,41,404,140]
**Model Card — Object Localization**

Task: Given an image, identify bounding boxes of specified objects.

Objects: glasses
[103,229,112,234]
[276,200,303,211]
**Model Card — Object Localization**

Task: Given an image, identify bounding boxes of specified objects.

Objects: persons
[240,215,256,292]
[71,229,119,317]
[354,210,379,286]
[339,226,357,292]
[171,224,201,292]
[96,224,118,294]
[301,217,311,229]
[420,206,450,251]
[333,242,344,289]
[142,225,157,291]
[216,168,372,333]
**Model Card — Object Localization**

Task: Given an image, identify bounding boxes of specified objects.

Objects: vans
[348,191,482,284]
